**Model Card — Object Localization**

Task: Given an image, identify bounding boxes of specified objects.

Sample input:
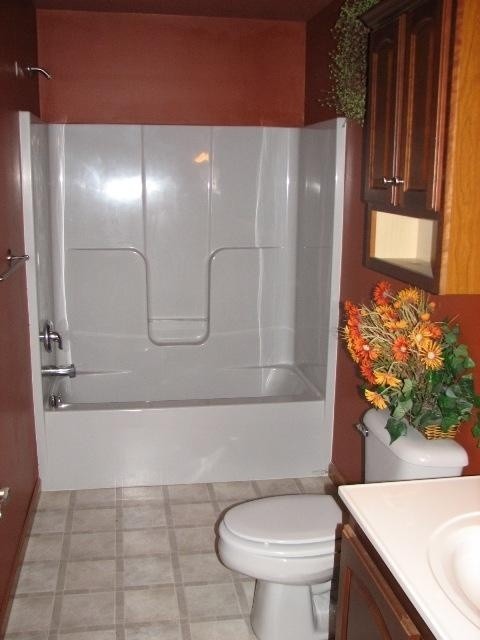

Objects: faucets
[42,364,49,376]
[49,362,76,379]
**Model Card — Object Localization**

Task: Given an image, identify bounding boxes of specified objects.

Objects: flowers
[339,280,479,450]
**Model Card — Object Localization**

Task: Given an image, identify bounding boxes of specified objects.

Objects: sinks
[428,510,480,630]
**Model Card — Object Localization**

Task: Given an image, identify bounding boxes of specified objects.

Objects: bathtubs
[44,364,330,493]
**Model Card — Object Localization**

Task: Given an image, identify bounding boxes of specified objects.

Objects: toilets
[216,406,469,639]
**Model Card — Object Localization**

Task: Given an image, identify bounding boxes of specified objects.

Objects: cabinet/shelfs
[335,517,437,640]
[356,0,480,296]
